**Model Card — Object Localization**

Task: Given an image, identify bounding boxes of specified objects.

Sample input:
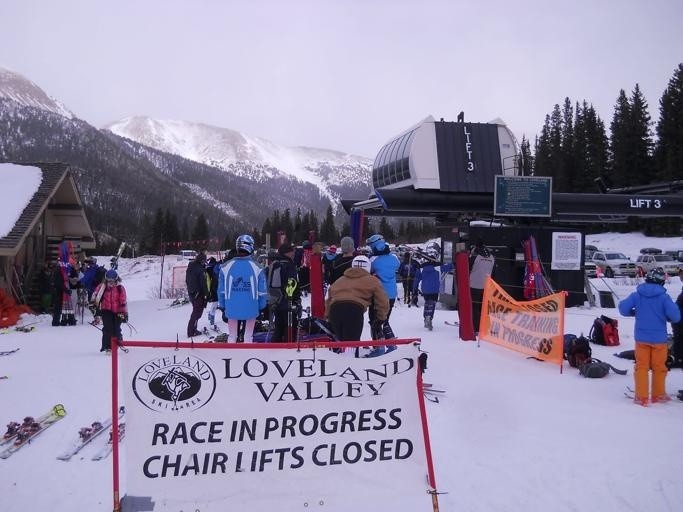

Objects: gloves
[256,311,264,320]
[222,312,227,323]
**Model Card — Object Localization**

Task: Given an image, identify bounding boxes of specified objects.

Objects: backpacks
[579,358,626,378]
[93,265,107,287]
[566,337,590,368]
[586,315,618,345]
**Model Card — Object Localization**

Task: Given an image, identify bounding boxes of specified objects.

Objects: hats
[197,253,206,263]
[327,245,336,252]
[340,236,355,253]
[85,257,96,263]
[278,243,294,255]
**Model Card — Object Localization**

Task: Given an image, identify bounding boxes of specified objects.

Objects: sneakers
[384,345,396,354]
[633,397,647,407]
[651,395,672,403]
[364,349,384,358]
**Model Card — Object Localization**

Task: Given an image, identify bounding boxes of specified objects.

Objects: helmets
[104,270,118,282]
[235,234,255,254]
[646,267,665,281]
[366,234,385,253]
[351,255,371,274]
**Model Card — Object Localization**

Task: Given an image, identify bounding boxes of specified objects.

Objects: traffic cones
[662,271,669,283]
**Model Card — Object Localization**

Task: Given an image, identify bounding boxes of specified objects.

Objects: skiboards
[56,242,78,325]
[94,241,125,306]
[399,242,456,275]
[1,404,65,461]
[525,233,544,302]
[56,407,123,461]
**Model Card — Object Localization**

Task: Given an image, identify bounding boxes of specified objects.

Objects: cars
[257,244,275,255]
[397,245,413,258]
[583,244,682,278]
[176,250,197,261]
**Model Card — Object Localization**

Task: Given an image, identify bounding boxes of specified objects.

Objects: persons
[671,268,683,368]
[618,267,681,406]
[39,254,105,326]
[185,233,457,357]
[468,238,496,332]
[93,270,128,351]
